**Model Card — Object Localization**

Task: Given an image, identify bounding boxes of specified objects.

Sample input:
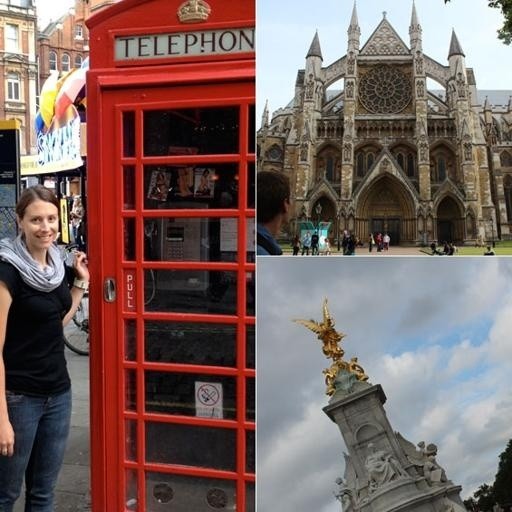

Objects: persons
[67,190,76,238]
[439,241,450,255]
[448,242,454,255]
[478,234,485,248]
[255,169,291,255]
[152,170,170,200]
[196,168,213,194]
[291,228,392,255]
[174,167,190,196]
[69,194,85,246]
[475,236,480,248]
[431,240,440,254]
[0,184,92,511]
[483,245,494,255]
[323,368,336,395]
[348,354,368,381]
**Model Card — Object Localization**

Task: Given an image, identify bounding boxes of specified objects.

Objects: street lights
[489,216,495,248]
[315,201,323,256]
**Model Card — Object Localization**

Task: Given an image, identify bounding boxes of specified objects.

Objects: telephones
[128,198,213,292]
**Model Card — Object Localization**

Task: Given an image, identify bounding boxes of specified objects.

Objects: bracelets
[72,278,90,290]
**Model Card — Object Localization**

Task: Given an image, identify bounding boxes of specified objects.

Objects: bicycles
[62,242,89,356]
